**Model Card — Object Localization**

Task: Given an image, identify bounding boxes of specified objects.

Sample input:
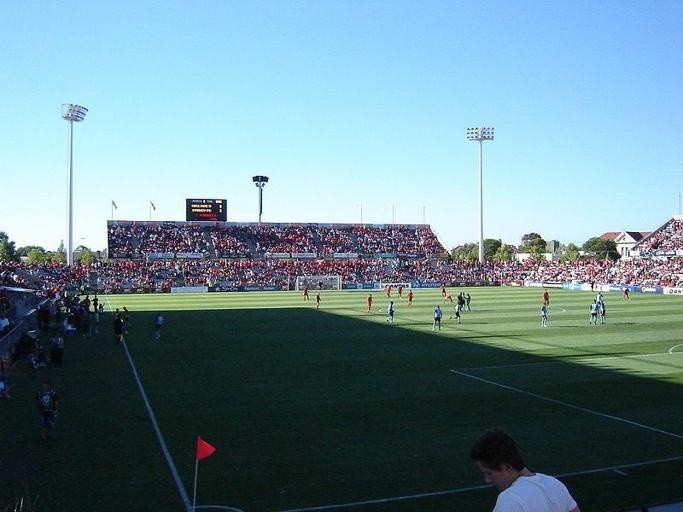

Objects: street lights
[60,101,89,270]
[466,124,495,264]
[251,175,268,223]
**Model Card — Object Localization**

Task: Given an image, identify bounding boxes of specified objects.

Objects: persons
[541,222,681,287]
[595,292,603,304]
[543,288,549,307]
[398,286,403,298]
[387,286,392,298]
[152,312,165,340]
[457,292,471,312]
[36,383,59,440]
[432,306,442,331]
[599,301,606,325]
[540,303,548,327]
[316,292,322,310]
[623,287,629,301]
[108,222,452,263]
[113,306,129,345]
[368,293,374,313]
[594,280,598,291]
[471,432,581,512]
[387,301,395,328]
[441,287,453,305]
[105,260,252,293]
[591,281,594,292]
[449,301,461,324]
[589,300,599,325]
[405,290,414,306]
[303,287,310,301]
[3,259,106,395]
[249,258,541,290]
